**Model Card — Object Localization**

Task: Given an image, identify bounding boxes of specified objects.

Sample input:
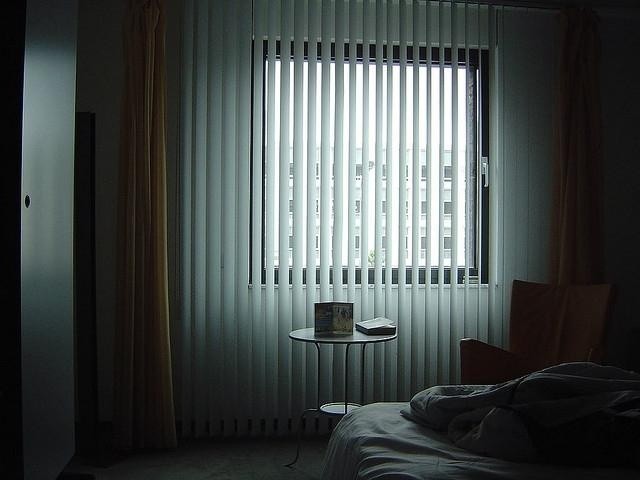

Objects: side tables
[283,326,406,468]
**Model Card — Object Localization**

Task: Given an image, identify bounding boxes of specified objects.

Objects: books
[314,301,354,337]
[355,316,396,336]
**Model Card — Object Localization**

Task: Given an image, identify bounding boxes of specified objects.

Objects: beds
[326,392,638,480]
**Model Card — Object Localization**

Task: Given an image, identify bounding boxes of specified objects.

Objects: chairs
[444,278,624,392]
